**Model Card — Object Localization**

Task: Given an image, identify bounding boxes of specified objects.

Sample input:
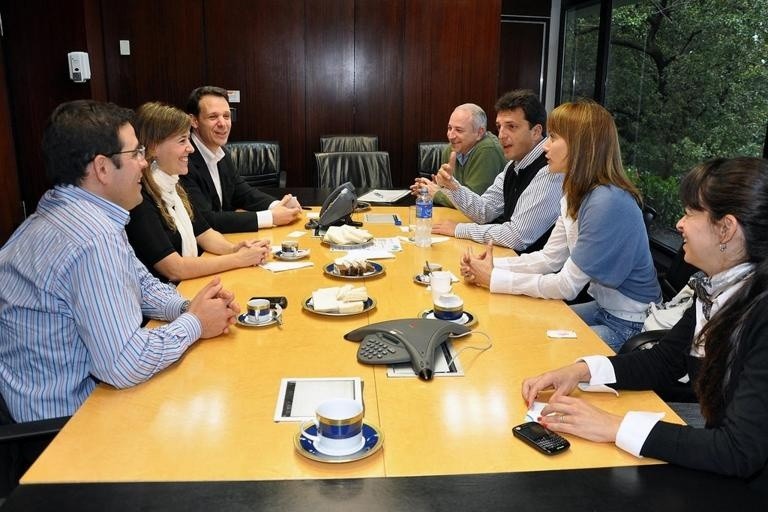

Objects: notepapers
[526,401,556,426]
[546,329,577,339]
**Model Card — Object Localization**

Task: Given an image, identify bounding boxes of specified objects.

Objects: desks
[1,189,768,512]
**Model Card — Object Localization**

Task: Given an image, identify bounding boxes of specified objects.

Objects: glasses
[106,144,146,161]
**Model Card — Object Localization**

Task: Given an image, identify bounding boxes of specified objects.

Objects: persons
[179,86,302,233]
[0,99,242,424]
[521,156,768,478]
[458,99,663,354]
[432,89,566,259]
[127,103,271,279]
[408,104,509,209]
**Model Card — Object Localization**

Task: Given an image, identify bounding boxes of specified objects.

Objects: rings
[558,415,564,424]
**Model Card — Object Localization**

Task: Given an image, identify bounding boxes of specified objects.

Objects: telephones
[305,181,358,230]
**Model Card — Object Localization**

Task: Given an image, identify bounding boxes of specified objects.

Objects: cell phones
[512,421,570,456]
[250,296,287,309]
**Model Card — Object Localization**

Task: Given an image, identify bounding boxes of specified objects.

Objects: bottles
[414,186,434,247]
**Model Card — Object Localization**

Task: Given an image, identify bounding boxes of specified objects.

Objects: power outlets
[227,91,240,102]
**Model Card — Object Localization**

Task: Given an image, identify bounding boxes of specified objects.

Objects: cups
[423,264,441,272]
[434,295,463,321]
[312,398,363,454]
[281,241,298,257]
[246,299,268,320]
[432,272,450,292]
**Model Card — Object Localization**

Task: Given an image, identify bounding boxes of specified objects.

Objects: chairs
[417,142,449,181]
[320,136,378,153]
[313,152,394,189]
[225,141,287,188]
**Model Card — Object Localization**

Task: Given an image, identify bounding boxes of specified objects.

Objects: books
[357,188,412,206]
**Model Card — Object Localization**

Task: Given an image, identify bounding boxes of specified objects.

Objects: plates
[273,249,310,257]
[417,306,477,325]
[322,235,375,245]
[411,272,429,284]
[323,261,384,278]
[301,294,376,315]
[293,418,383,462]
[237,312,281,326]
